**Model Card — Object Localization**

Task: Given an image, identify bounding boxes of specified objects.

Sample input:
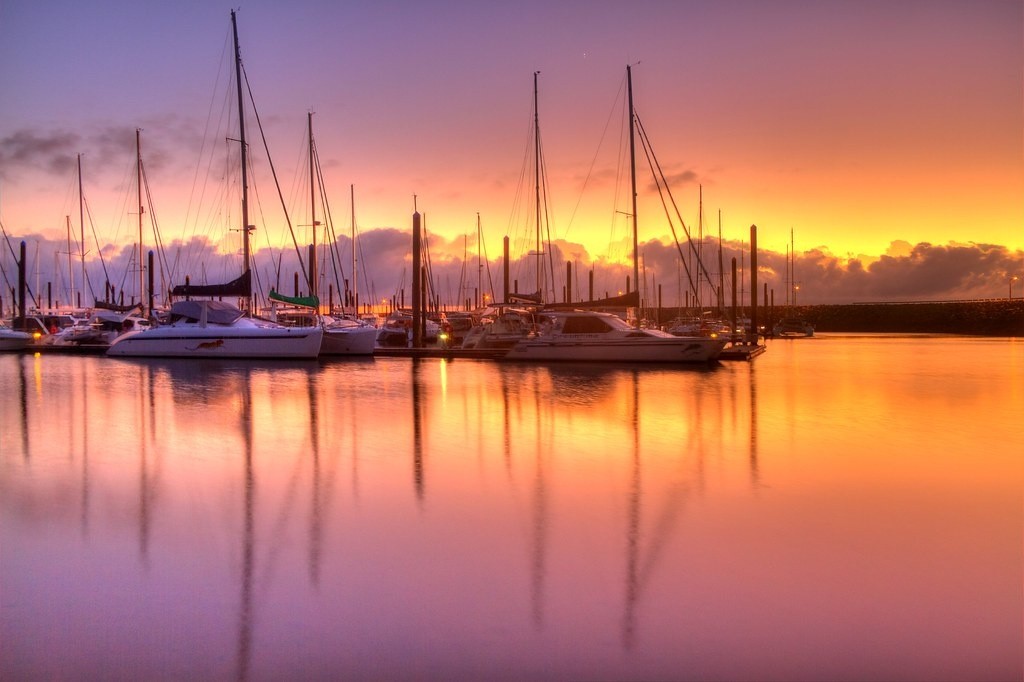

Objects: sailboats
[104,4,326,362]
[1,60,819,361]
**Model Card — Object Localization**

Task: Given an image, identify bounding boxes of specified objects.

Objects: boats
[494,307,734,365]
